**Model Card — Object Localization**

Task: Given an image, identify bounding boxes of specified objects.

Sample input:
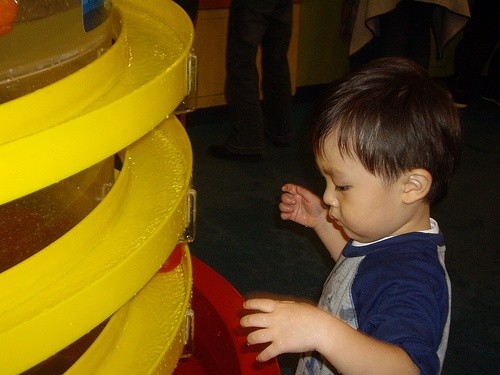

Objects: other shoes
[449,81,472,107]
[480,89,500,107]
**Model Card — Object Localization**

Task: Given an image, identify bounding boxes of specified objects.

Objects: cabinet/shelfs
[287,0,346,97]
[186,9,265,110]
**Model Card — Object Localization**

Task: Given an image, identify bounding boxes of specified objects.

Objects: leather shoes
[208,143,263,160]
[262,130,295,145]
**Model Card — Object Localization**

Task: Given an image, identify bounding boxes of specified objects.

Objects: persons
[237,54,464,375]
[339,0,471,76]
[208,0,298,162]
[443,0,500,110]
